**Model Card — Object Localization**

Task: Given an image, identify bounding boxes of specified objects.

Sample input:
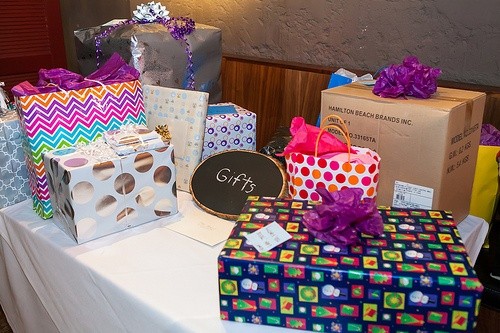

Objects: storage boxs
[217,195,484,333]
[200,102,256,160]
[74,19,222,104]
[320,80,486,227]
[0,111,32,209]
[41,124,178,244]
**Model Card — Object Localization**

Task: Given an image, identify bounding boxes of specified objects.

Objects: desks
[0,189,490,333]
